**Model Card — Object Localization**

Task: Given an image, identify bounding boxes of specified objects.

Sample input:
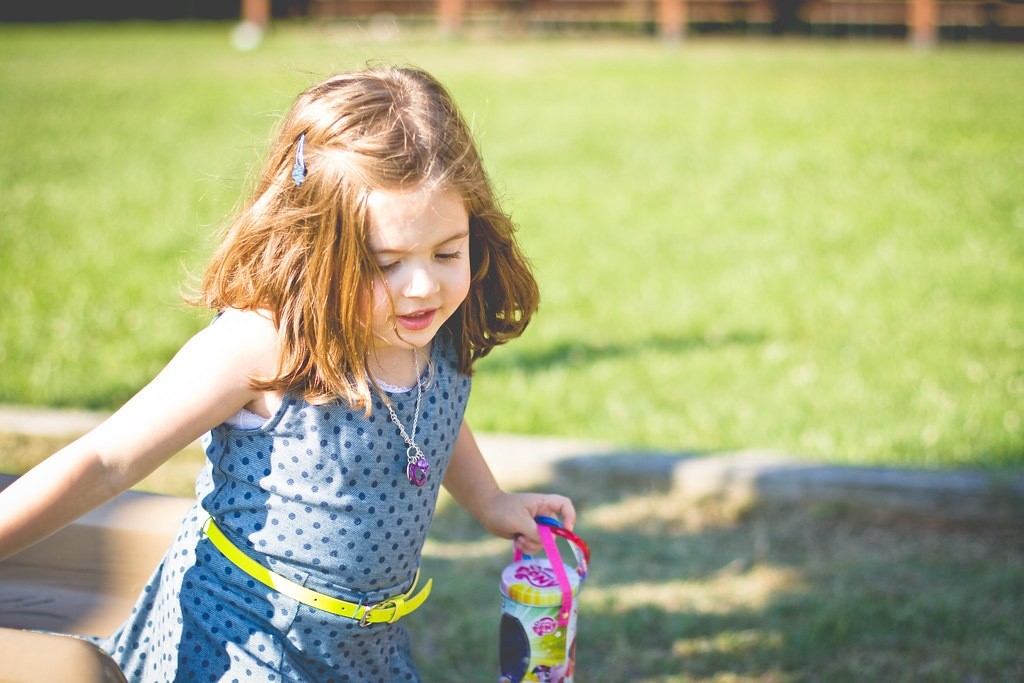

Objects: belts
[193,503,433,627]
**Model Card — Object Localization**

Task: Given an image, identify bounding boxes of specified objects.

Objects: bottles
[498,558,579,682]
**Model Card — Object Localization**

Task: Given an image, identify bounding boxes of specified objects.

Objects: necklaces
[370,348,429,486]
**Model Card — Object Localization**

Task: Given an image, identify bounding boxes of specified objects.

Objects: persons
[0,68,577,683]
